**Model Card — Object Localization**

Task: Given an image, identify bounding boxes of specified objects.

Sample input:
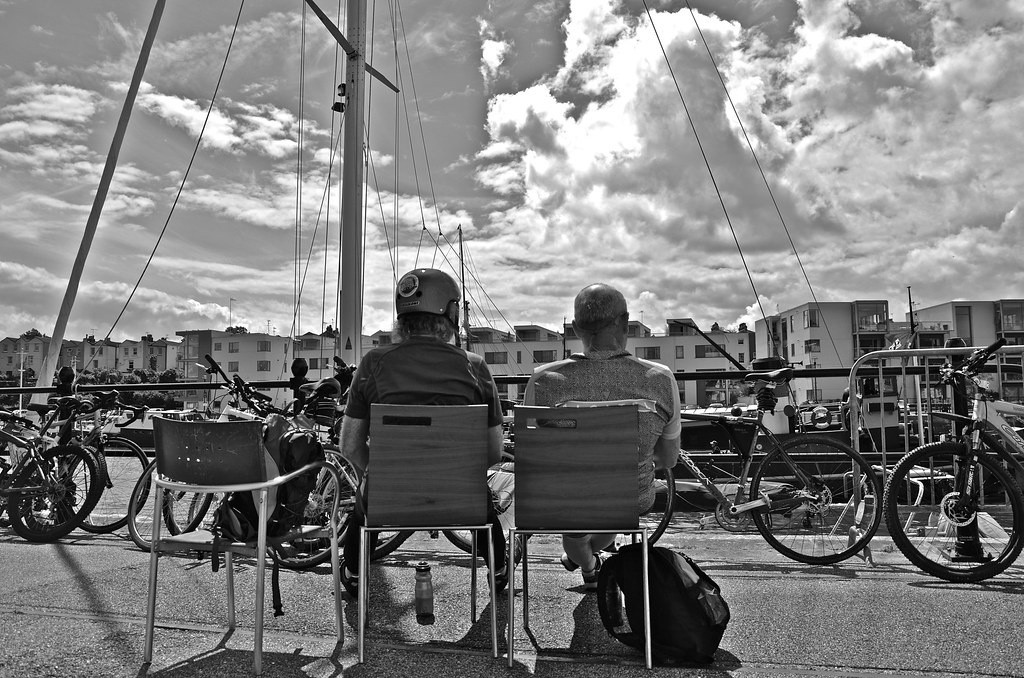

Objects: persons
[339,267,524,601]
[522,282,682,588]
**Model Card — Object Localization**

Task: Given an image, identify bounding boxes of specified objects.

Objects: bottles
[414,561,434,614]
[606,576,623,626]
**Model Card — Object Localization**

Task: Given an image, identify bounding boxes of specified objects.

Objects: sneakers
[582,552,612,591]
[331,547,358,597]
[487,535,522,593]
[560,553,580,571]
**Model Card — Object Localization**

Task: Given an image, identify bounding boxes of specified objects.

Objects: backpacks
[211,413,325,543]
[596,542,730,665]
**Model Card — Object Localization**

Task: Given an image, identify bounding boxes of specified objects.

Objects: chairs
[355,403,499,665]
[144,413,346,676]
[505,402,654,669]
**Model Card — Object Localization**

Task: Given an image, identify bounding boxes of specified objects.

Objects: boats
[20,0,1023,511]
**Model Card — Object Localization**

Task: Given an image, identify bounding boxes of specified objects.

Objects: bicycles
[883,338,1024,583]
[0,353,535,569]
[599,368,883,566]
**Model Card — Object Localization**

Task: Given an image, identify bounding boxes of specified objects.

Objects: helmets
[396,268,461,325]
